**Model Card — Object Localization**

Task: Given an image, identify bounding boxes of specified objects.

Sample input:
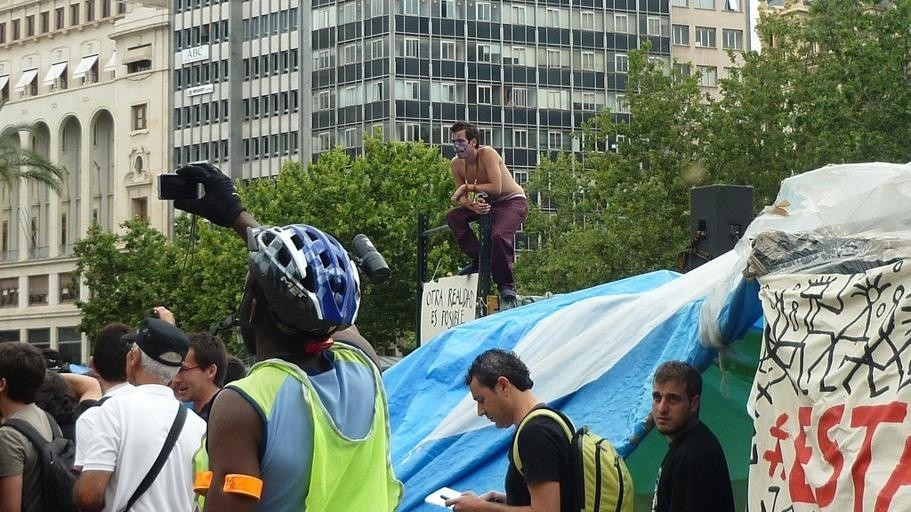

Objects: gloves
[172,159,247,229]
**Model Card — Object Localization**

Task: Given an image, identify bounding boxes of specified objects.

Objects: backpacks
[0,409,81,512]
[513,407,635,512]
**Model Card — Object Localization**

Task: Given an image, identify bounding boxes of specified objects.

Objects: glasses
[178,365,201,375]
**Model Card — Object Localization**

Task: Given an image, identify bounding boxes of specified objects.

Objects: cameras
[156,173,201,201]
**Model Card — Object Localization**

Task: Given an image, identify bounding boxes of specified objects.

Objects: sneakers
[499,286,517,299]
[457,260,479,276]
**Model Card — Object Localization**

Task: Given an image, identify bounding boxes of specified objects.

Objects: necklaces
[464,149,479,204]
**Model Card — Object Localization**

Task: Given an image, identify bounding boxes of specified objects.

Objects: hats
[119,316,190,367]
[246,224,363,341]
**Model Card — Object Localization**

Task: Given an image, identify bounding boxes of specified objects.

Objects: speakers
[689,184,753,272]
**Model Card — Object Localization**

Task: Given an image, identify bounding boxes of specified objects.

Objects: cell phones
[440,494,450,500]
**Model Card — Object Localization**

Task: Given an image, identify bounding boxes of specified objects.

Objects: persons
[445,119,530,303]
[173,160,405,511]
[651,360,735,511]
[445,347,582,512]
